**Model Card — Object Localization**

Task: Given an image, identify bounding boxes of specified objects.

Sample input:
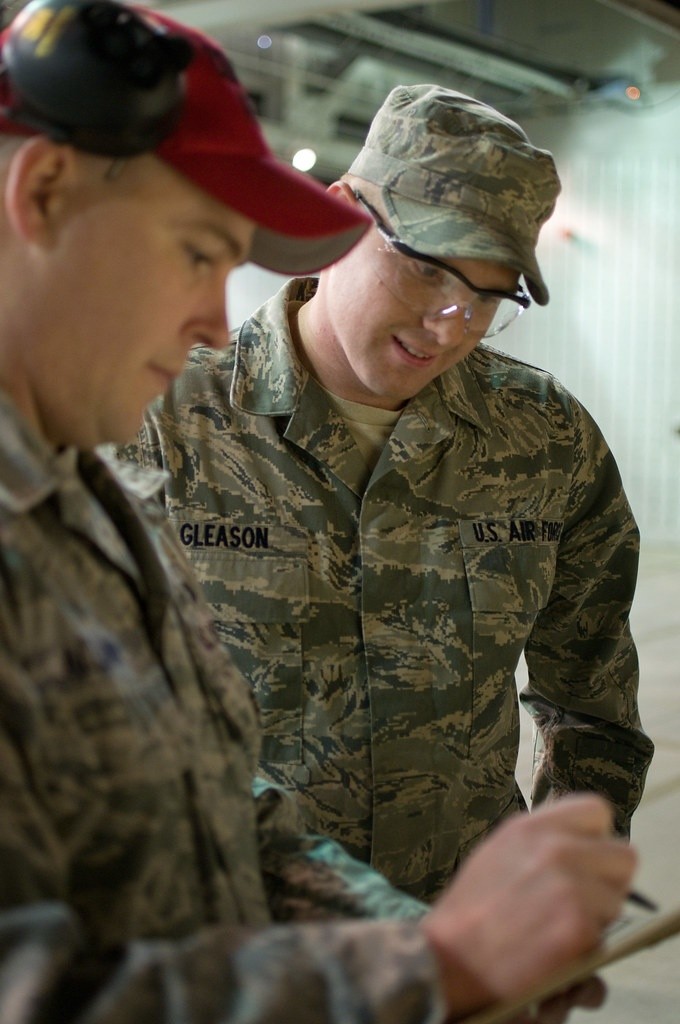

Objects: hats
[348,83,562,306]
[1,0,375,276]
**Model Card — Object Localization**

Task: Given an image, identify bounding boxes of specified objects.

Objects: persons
[114,84,656,925]
[0,1,636,1024]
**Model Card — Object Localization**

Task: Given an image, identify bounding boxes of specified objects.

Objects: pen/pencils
[627,890,661,914]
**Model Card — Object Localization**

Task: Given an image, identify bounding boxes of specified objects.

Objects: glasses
[354,190,530,338]
